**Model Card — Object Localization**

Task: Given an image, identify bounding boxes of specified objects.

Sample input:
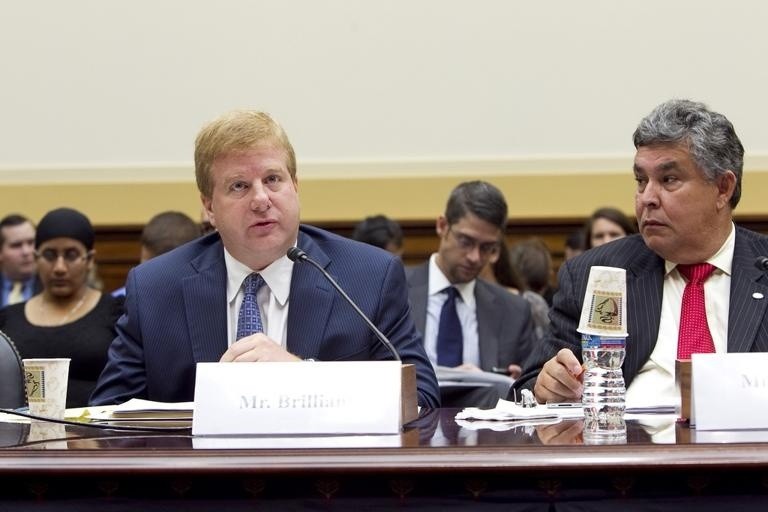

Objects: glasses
[448,229,497,254]
[30,249,91,264]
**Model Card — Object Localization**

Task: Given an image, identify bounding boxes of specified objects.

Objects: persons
[504,233,553,303]
[1,207,126,411]
[88,109,443,418]
[507,99,767,415]
[583,206,637,251]
[535,419,584,445]
[66,421,192,450]
[560,226,583,262]
[108,211,201,301]
[350,213,406,262]
[414,408,531,445]
[406,181,540,410]
[0,214,37,314]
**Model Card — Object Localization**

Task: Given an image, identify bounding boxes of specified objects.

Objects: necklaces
[37,292,88,327]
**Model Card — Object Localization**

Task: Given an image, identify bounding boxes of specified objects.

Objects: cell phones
[491,366,512,376]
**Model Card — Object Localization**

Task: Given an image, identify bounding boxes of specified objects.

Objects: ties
[7,281,23,304]
[437,286,461,367]
[677,263,716,359]
[237,273,265,342]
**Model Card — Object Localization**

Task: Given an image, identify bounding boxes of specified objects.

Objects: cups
[26,421,69,451]
[576,267,629,338]
[20,357,71,421]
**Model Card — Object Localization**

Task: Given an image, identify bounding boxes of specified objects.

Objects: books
[111,397,193,433]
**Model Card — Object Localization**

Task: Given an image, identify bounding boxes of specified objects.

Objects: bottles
[580,329,627,444]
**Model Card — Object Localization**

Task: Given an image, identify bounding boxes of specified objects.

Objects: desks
[1,407,767,512]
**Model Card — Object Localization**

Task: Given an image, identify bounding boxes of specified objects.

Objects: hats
[35,209,94,250]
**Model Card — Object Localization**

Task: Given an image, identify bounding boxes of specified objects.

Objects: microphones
[287,246,401,360]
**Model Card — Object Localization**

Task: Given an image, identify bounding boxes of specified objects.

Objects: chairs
[0,407,29,449]
[1,327,33,409]
